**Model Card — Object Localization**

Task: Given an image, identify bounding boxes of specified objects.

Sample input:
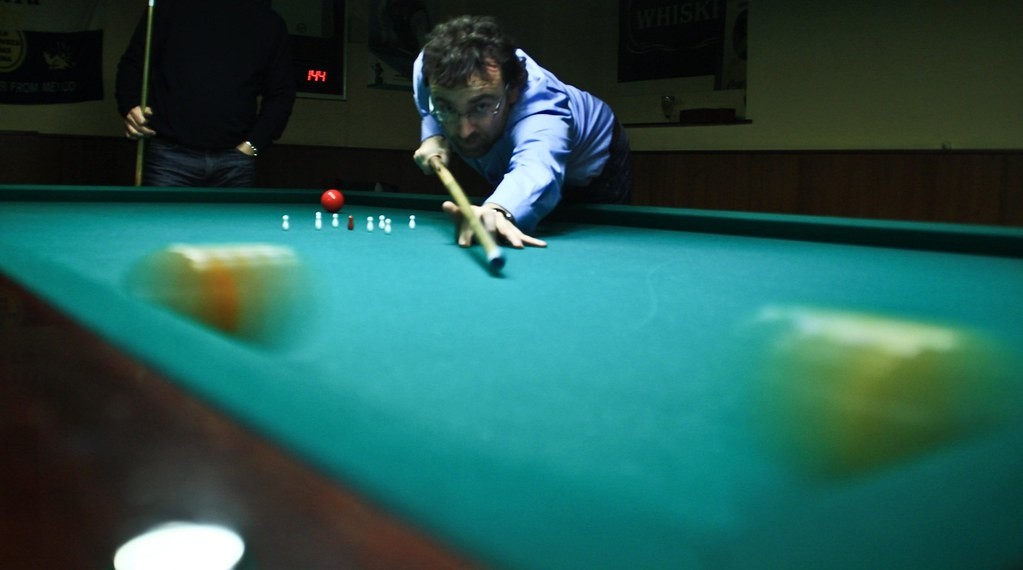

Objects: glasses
[429,82,511,122]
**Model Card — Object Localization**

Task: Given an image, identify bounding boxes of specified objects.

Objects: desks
[0,183,1023,569]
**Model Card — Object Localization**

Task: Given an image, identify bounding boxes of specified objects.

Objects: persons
[411,14,634,248]
[115,0,299,188]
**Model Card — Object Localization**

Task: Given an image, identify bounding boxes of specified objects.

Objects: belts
[610,113,620,144]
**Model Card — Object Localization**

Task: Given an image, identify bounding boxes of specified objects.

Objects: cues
[429,155,506,269]
[135,0,156,189]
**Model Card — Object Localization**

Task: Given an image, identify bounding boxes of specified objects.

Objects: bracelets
[246,141,258,155]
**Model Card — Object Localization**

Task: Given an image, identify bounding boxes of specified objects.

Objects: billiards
[320,189,344,212]
